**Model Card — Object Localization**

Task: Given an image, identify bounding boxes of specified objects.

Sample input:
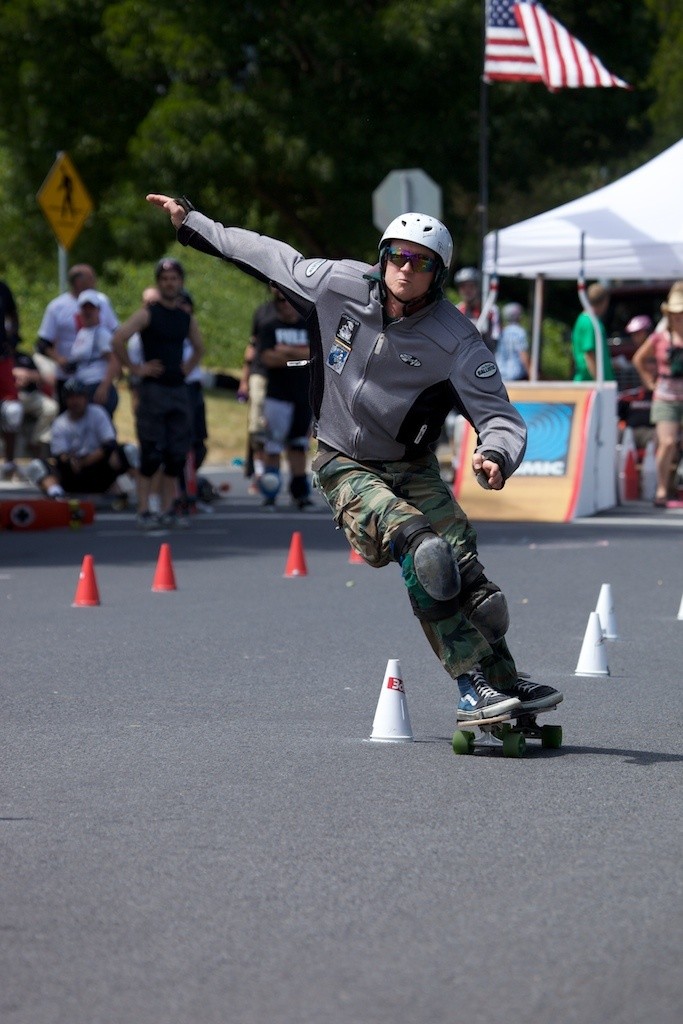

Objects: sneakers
[457,661,521,721]
[496,672,564,711]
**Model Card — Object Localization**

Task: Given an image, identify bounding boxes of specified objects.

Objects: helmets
[155,258,185,283]
[378,213,452,288]
[454,266,482,287]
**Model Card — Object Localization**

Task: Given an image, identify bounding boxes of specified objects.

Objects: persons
[569,283,613,381]
[488,302,540,381]
[0,264,140,505]
[441,267,502,486]
[146,194,563,758]
[111,257,215,529]
[609,282,683,508]
[235,285,316,512]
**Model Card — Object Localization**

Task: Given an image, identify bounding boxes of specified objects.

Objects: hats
[77,289,100,308]
[659,281,683,313]
[59,376,89,398]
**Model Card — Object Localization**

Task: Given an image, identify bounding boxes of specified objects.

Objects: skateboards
[451,702,564,757]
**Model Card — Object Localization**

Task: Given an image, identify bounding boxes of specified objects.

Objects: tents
[482,138,683,337]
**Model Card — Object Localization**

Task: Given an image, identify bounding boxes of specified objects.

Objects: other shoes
[654,487,669,506]
[137,499,216,530]
[294,499,320,515]
[258,498,278,514]
[249,474,265,498]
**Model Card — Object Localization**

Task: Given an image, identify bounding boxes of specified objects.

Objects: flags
[484,0,631,92]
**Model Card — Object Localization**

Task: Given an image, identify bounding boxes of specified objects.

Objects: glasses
[384,246,439,274]
